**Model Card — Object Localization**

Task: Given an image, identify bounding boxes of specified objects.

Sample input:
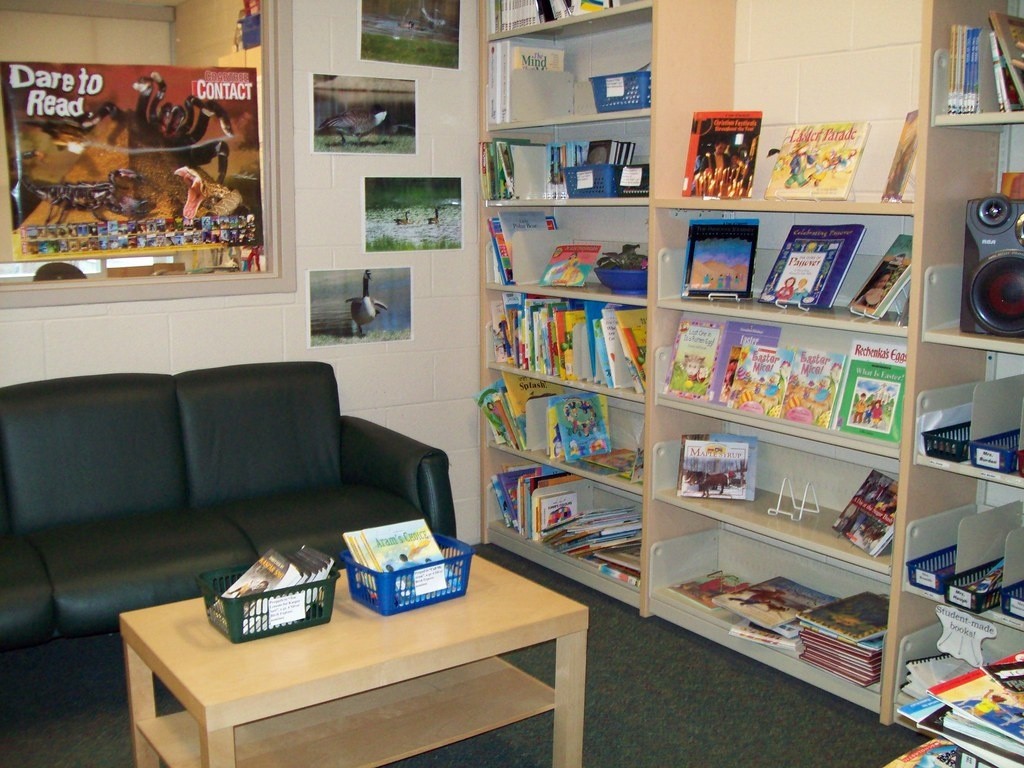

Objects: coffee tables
[118,546,590,768]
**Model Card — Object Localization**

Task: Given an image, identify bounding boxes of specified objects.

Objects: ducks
[393,210,411,225]
[398,21,415,30]
[426,206,441,224]
[317,104,387,145]
[345,269,388,336]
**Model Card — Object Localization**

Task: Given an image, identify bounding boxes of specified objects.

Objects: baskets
[1015,450,1024,478]
[920,420,971,463]
[339,532,476,616]
[563,163,620,198]
[1000,580,1024,620]
[968,430,1021,473]
[942,557,1004,614]
[906,543,957,596]
[589,71,651,114]
[197,555,341,644]
[614,164,649,197]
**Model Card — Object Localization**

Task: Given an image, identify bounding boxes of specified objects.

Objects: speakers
[959,194,1024,339]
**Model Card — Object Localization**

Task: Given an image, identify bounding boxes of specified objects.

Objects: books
[709,321,781,409]
[681,439,748,499]
[676,434,757,501]
[835,339,907,444]
[883,652,1024,768]
[782,345,846,429]
[343,518,461,608]
[882,109,919,202]
[681,218,760,299]
[482,2,652,589]
[831,469,898,557]
[666,572,752,611]
[946,12,1024,116]
[846,234,912,318]
[727,344,794,420]
[712,577,888,689]
[765,121,871,201]
[757,224,866,308]
[683,110,763,199]
[663,317,724,401]
[208,545,335,635]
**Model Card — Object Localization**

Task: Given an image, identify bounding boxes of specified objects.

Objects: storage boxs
[194,562,341,644]
[339,533,478,617]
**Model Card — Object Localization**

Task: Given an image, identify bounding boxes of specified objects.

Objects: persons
[858,270,892,309]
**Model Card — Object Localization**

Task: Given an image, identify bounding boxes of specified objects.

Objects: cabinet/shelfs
[651,196,923,727]
[478,0,651,619]
[878,2,1024,768]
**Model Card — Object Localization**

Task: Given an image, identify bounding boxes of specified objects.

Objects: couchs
[0,361,457,655]
